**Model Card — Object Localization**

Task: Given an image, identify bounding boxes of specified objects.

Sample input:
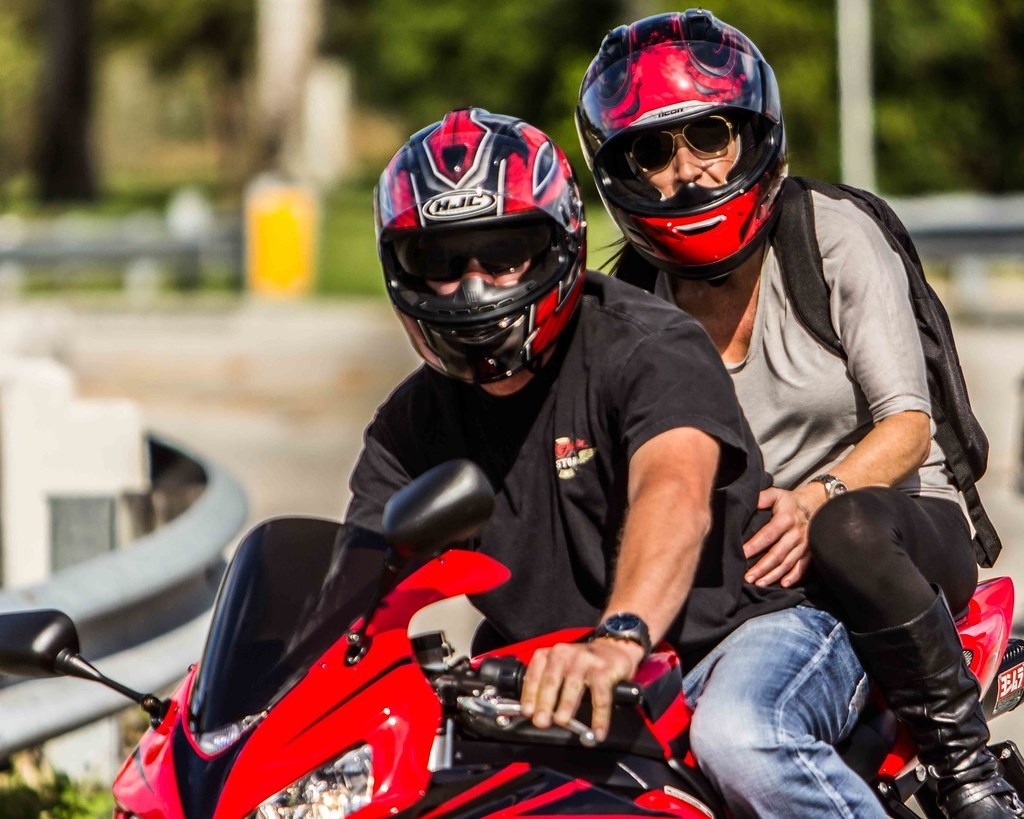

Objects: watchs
[587,612,652,665]
[811,474,848,499]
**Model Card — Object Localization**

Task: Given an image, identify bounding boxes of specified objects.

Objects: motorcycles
[1,455,1024,819]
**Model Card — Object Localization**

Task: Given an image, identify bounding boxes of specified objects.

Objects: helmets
[371,106,593,390]
[573,8,788,288]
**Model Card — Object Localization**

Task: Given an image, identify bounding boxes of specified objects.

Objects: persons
[288,107,887,819]
[574,9,1024,819]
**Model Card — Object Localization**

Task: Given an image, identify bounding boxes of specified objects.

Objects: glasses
[627,115,734,174]
[392,227,553,282]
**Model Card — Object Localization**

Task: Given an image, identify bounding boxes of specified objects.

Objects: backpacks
[769,176,1008,571]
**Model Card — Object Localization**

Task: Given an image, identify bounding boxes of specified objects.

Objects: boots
[846,582,1024,818]
